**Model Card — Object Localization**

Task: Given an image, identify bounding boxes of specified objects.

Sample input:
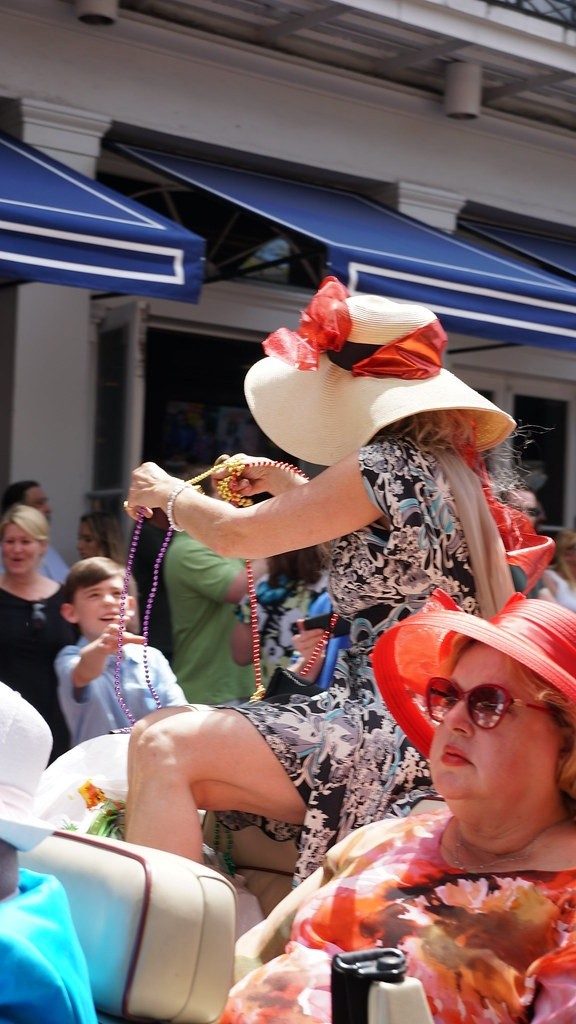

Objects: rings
[124,500,134,510]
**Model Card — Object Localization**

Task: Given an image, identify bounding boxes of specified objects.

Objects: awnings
[0,133,206,306]
[113,140,576,352]
[459,221,576,278]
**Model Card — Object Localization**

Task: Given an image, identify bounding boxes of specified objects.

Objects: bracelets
[167,484,192,532]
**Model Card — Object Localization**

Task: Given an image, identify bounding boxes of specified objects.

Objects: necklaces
[186,460,339,703]
[215,821,236,877]
[115,507,174,727]
[455,841,530,871]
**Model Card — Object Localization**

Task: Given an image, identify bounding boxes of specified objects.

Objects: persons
[234,547,330,691]
[542,528,576,609]
[1,505,77,769]
[165,466,267,706]
[219,597,576,1023]
[503,485,546,529]
[78,510,139,635]
[0,481,71,586]
[56,557,187,748]
[124,296,553,902]
[0,683,100,1024]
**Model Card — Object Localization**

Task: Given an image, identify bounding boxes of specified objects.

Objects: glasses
[510,505,541,517]
[426,676,560,730]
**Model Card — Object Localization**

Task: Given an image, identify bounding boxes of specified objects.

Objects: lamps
[442,64,485,119]
[76,0,117,26]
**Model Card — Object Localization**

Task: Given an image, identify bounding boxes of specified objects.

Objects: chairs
[18,828,238,1024]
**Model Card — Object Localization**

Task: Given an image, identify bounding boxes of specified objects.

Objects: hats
[0,678,53,851]
[243,276,518,468]
[373,589,576,757]
